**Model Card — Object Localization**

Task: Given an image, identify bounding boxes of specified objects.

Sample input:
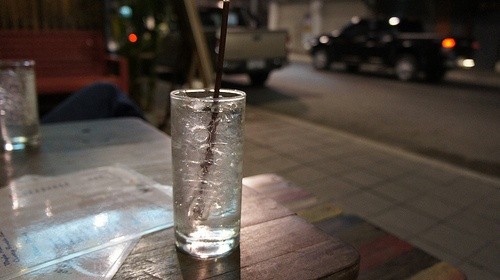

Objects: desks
[0,116,361,280]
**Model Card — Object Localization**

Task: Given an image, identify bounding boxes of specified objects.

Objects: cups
[170,88,245,257]
[1,60,40,151]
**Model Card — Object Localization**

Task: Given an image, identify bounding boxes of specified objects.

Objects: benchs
[0,29,130,103]
[239,171,468,280]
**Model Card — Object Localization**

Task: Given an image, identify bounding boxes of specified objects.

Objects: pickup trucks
[155,5,291,89]
[308,17,482,86]
